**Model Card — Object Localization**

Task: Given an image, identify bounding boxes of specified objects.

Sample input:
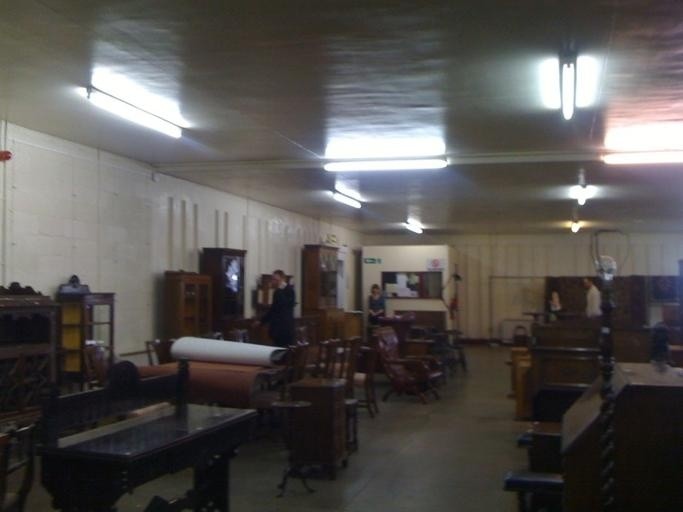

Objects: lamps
[572,217,579,233]
[404,220,423,234]
[600,151,681,164]
[559,33,577,119]
[322,157,449,172]
[578,170,587,206]
[328,187,362,210]
[86,86,183,139]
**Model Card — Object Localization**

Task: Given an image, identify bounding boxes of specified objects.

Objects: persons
[583,276,604,318]
[367,283,385,326]
[251,269,296,347]
[548,291,561,311]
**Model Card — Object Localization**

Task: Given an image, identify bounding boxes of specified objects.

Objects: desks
[33,404,258,512]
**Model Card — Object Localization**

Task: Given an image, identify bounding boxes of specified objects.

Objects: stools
[272,399,317,497]
[289,377,347,481]
[345,399,358,454]
[501,471,564,512]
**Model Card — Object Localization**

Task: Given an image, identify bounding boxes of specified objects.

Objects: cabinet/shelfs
[203,248,247,332]
[0,282,61,435]
[304,244,339,314]
[164,271,212,338]
[56,275,116,392]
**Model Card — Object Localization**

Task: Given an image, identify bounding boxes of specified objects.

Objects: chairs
[0,422,36,512]
[371,329,442,405]
[310,337,340,377]
[335,335,364,420]
[354,344,380,418]
[146,340,170,365]
